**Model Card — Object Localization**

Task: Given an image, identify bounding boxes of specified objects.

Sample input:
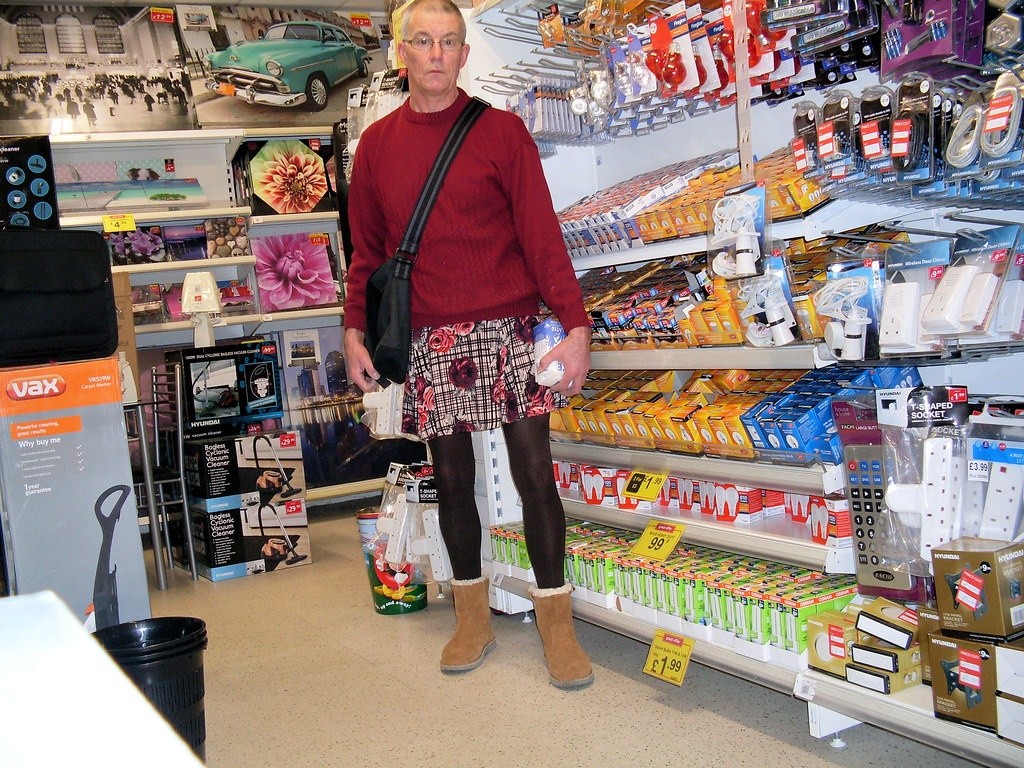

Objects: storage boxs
[489,147,1024,748]
[157,340,312,582]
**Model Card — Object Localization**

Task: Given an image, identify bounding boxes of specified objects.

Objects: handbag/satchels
[0,223,121,368]
[367,256,412,385]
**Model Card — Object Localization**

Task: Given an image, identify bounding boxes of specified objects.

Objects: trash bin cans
[356,506,428,615]
[90,615,209,767]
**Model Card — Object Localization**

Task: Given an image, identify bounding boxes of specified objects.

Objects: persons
[341,0,595,690]
[2,71,189,126]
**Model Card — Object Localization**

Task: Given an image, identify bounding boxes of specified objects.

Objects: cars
[202,20,374,113]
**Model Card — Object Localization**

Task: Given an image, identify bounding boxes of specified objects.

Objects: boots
[440,575,496,672]
[528,579,592,687]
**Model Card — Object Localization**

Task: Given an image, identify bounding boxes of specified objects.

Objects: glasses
[403,38,466,50]
